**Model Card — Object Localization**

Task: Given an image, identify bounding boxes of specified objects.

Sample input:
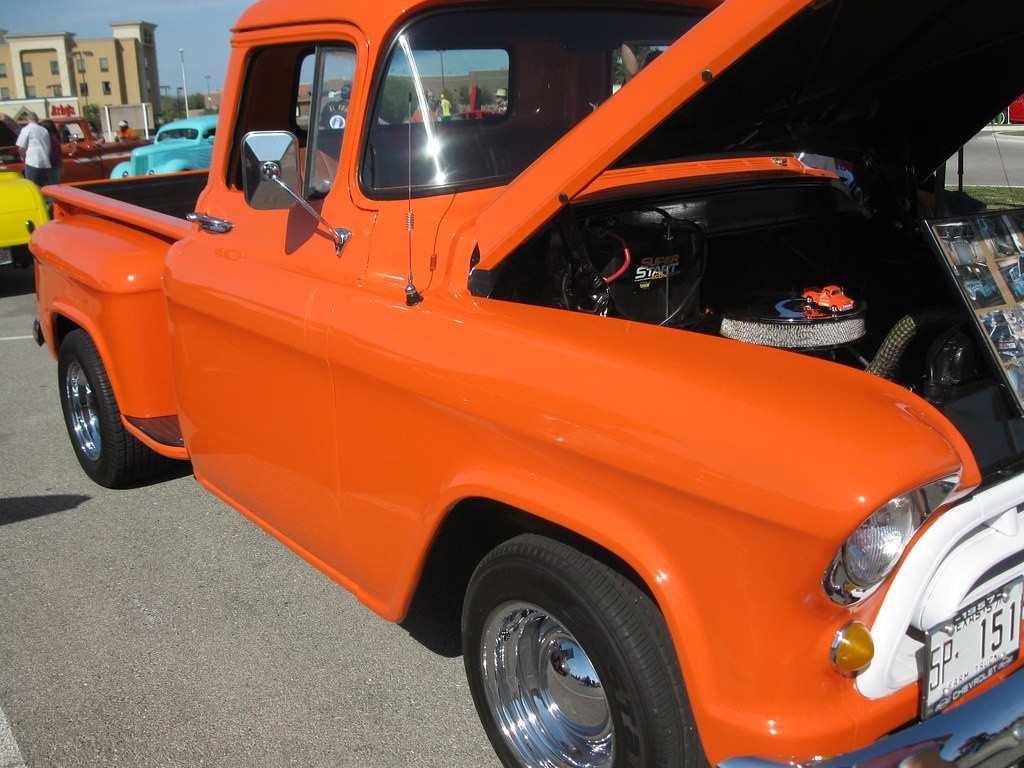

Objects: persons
[87,120,105,144]
[39,118,63,184]
[15,111,52,202]
[491,88,508,110]
[321,85,351,130]
[412,89,452,122]
[114,119,139,141]
[620,44,664,87]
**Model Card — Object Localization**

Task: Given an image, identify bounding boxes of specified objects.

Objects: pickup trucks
[0,114,149,186]
[29,0,1024,766]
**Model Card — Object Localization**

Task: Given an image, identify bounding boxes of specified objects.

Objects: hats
[494,89,507,96]
[441,94,444,98]
[118,121,128,127]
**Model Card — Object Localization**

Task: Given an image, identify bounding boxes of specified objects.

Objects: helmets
[340,86,352,99]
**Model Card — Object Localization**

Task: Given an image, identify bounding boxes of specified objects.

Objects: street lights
[67,51,93,106]
[205,76,212,108]
[176,87,183,119]
[438,49,446,92]
[179,49,190,119]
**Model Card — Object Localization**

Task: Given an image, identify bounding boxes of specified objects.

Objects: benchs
[249,126,571,210]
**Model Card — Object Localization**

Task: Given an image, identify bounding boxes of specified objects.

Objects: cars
[992,92,1024,124]
[109,113,221,180]
[0,165,50,279]
